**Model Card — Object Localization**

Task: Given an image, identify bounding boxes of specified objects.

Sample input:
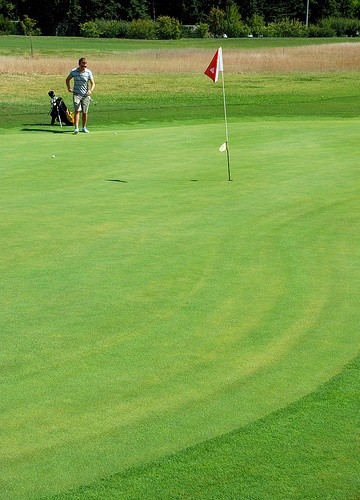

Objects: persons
[66,58,95,134]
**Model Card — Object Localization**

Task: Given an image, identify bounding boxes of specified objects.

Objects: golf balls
[114,133,116,135]
[52,156,55,158]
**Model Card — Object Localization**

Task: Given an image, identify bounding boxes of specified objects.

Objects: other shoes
[82,128,89,133]
[74,129,79,134]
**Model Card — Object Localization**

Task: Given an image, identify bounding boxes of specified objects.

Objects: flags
[203,47,224,82]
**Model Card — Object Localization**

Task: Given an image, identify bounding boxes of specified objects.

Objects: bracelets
[90,89,93,92]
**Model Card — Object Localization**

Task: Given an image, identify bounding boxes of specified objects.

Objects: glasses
[81,66,87,68]
